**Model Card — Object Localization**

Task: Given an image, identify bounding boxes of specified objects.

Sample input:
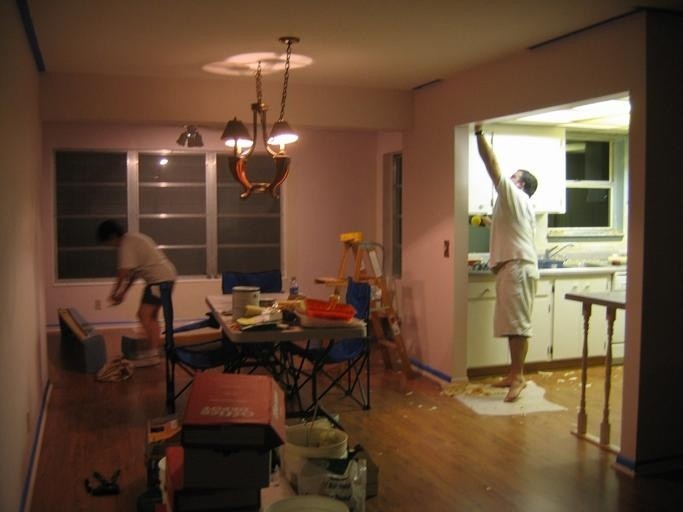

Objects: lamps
[176,124,204,149]
[220,34,301,200]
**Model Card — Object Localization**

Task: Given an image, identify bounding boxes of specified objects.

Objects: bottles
[290,277,299,300]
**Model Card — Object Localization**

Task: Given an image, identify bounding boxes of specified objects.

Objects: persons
[97,218,177,359]
[473,123,539,401]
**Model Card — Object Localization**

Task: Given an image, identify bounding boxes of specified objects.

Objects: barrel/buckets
[233,286,260,321]
[280,427,348,494]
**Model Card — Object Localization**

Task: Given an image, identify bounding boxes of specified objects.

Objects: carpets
[454,379,568,417]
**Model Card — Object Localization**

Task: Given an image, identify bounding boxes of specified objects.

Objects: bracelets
[476,130,483,136]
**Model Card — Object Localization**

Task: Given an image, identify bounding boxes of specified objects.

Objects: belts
[490,261,512,275]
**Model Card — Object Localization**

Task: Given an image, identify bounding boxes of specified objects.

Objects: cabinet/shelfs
[468,121,566,215]
[466,272,627,377]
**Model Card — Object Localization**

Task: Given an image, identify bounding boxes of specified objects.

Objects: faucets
[545,243,574,258]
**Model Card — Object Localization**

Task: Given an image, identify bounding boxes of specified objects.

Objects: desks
[563,289,627,454]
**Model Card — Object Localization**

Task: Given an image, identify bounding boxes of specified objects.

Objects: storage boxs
[162,364,287,512]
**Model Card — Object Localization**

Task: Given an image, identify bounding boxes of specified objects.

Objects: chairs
[160,269,372,414]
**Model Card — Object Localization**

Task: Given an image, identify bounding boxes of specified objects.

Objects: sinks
[562,263,605,268]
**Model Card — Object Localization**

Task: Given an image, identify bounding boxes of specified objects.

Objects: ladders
[320,232,416,381]
